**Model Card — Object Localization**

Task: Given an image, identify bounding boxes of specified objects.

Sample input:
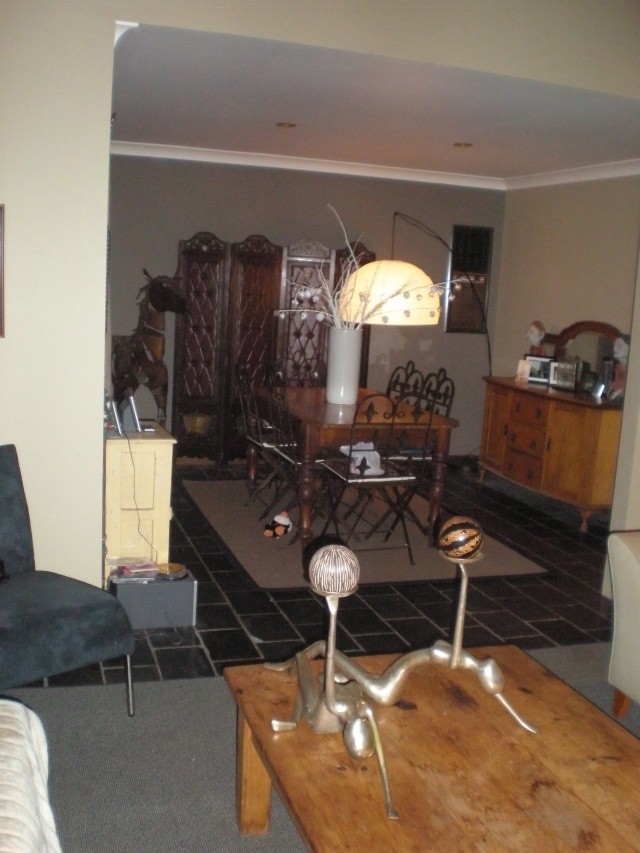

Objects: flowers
[276,201,475,328]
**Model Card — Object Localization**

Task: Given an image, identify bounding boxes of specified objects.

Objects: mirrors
[541,321,621,397]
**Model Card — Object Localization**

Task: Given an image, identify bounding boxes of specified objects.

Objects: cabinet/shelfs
[477,376,624,532]
[103,421,177,591]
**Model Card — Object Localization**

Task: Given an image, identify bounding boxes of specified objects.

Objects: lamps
[339,211,491,376]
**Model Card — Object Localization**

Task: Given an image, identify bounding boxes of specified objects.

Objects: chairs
[240,360,454,564]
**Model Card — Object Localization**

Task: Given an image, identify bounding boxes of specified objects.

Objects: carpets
[0,645,640,853]
[184,481,547,587]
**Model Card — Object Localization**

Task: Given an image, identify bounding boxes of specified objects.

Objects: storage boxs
[109,569,197,629]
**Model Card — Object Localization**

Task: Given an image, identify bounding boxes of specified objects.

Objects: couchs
[607,532,640,718]
[0,445,136,853]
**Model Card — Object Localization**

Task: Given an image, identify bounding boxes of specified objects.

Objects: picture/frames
[524,355,576,386]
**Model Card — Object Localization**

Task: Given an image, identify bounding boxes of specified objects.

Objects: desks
[264,386,461,551]
[225,645,640,853]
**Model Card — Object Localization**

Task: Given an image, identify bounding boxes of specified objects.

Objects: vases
[326,327,362,405]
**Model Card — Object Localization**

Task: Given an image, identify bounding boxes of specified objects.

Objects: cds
[157,562,186,580]
